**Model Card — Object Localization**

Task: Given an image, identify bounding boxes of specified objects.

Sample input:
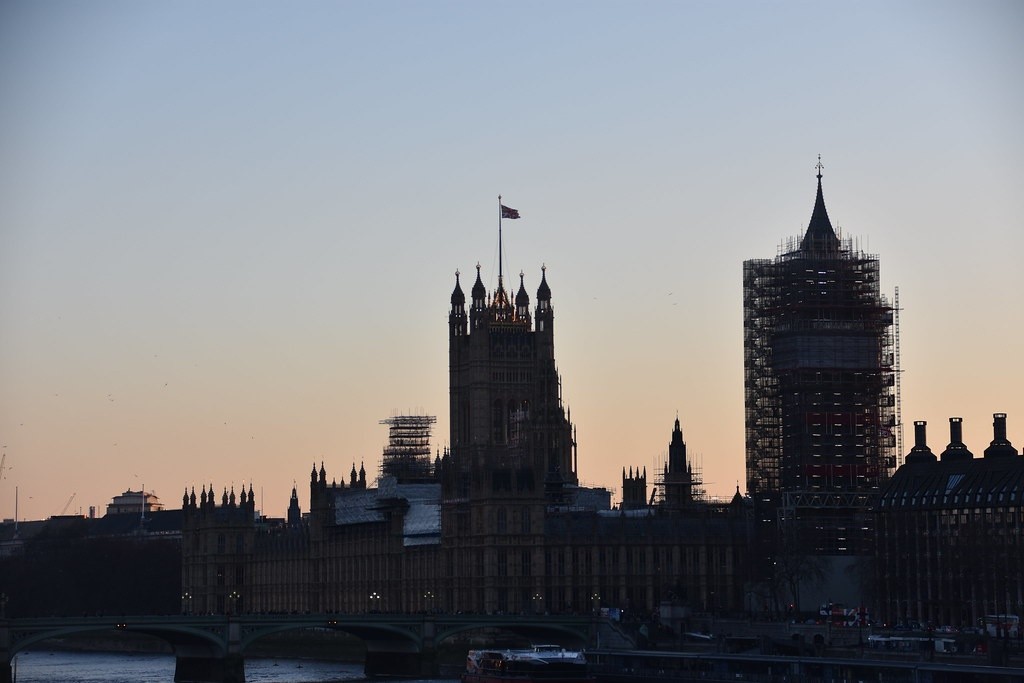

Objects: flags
[501,204,520,219]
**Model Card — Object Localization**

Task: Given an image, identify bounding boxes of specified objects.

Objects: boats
[463,643,587,683]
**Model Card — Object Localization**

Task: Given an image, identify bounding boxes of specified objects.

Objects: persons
[370,608,549,616]
[198,609,310,616]
